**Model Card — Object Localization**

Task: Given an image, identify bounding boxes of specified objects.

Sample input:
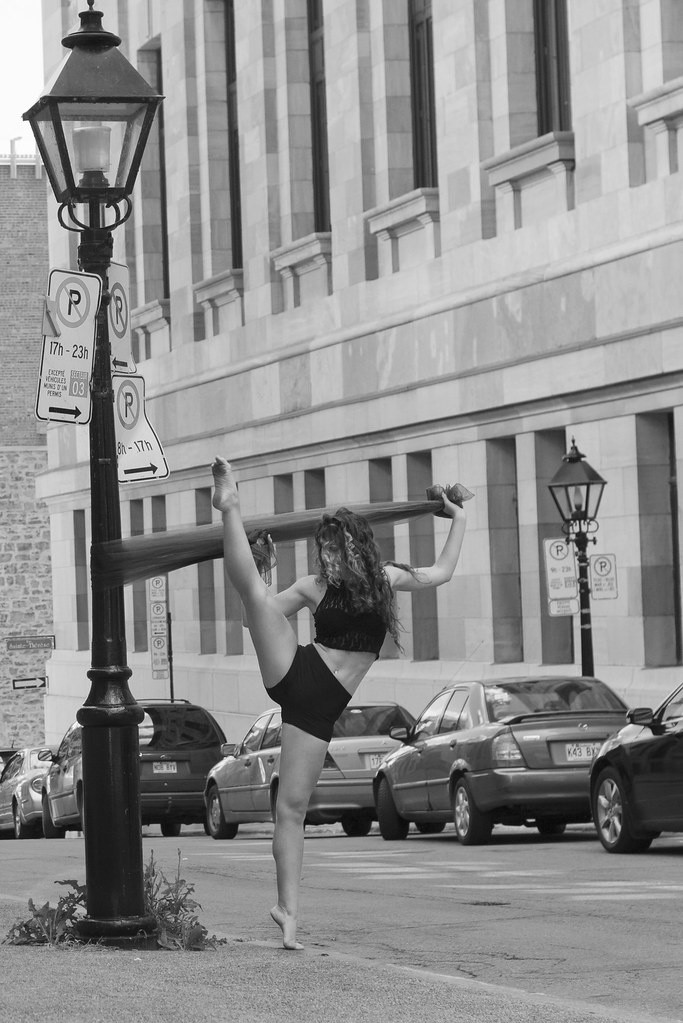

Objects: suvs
[36,697,226,840]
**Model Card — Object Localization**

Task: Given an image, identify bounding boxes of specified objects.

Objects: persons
[207,457,466,950]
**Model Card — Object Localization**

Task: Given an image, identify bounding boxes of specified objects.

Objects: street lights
[547,434,608,676]
[20,2,167,950]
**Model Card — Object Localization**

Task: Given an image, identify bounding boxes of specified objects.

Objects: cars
[590,679,683,854]
[202,700,417,839]
[0,743,63,840]
[372,674,631,847]
